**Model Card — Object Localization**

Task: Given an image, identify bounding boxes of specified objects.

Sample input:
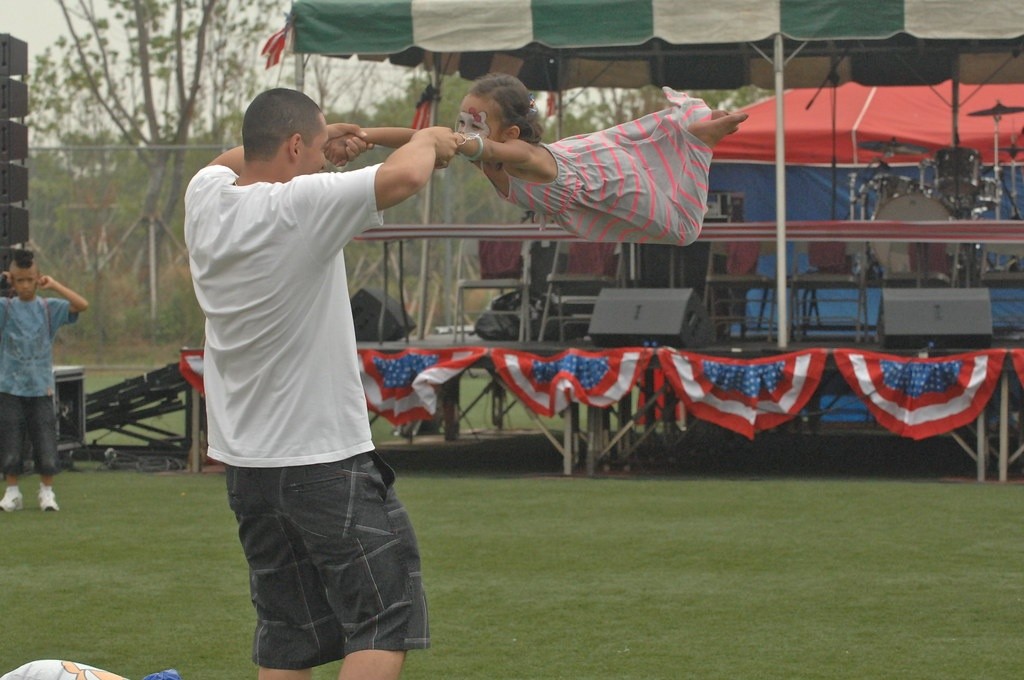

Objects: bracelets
[461,132,482,162]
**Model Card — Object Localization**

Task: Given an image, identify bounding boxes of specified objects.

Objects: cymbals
[966,104,1024,117]
[992,143,1024,155]
[856,140,929,156]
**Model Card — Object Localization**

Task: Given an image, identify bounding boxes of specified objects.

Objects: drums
[888,176,933,200]
[958,176,1003,210]
[869,191,957,276]
[936,146,982,198]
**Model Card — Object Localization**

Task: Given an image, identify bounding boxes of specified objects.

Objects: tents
[696,78,1024,339]
[284,0,1024,350]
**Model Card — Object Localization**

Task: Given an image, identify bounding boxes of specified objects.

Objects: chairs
[452,240,532,343]
[789,241,868,344]
[538,242,625,342]
[703,243,776,343]
[875,241,962,345]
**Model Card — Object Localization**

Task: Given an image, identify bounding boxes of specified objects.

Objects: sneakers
[0,486,25,512]
[37,483,59,512]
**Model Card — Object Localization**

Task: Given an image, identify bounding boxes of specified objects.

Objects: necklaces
[495,160,504,170]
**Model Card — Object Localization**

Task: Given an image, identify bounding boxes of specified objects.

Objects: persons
[316,70,749,250]
[183,87,461,680]
[0,249,88,511]
